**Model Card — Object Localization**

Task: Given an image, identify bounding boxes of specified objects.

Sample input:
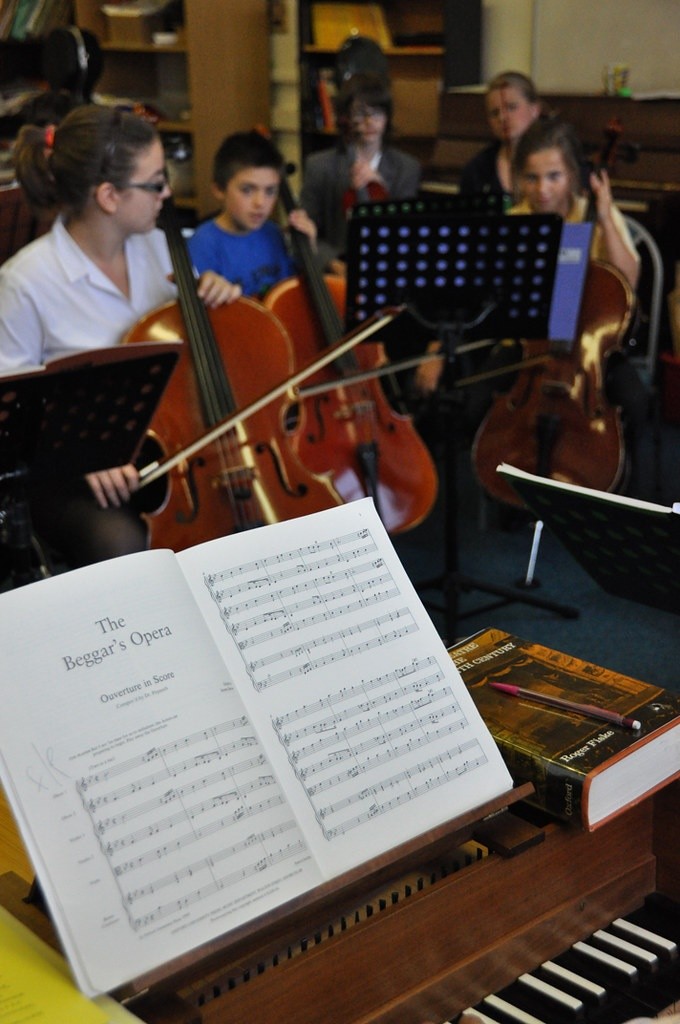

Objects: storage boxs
[107,13,165,45]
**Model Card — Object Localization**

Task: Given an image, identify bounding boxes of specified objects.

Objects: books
[446,628,679,833]
[0,496,514,1000]
[0,0,72,40]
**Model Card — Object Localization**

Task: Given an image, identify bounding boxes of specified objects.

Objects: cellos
[470,60,640,591]
[116,99,349,552]
[248,121,440,538]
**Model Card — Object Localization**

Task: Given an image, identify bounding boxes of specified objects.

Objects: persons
[298,79,424,274]
[508,118,641,390]
[0,105,241,563]
[461,73,549,217]
[187,128,318,301]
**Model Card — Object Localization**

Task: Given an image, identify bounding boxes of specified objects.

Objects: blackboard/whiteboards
[529,0,680,101]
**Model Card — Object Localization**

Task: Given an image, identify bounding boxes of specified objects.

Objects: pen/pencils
[489,682,642,730]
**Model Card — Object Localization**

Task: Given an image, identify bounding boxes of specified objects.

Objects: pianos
[0,623,680,1024]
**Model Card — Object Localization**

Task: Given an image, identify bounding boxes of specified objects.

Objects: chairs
[622,216,664,384]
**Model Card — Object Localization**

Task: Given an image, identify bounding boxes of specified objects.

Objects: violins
[341,131,393,223]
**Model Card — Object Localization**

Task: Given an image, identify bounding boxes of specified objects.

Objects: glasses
[121,177,170,193]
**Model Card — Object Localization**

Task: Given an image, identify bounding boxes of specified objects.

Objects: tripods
[342,212,584,648]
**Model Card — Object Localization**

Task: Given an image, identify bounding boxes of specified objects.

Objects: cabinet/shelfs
[74,0,268,217]
[296,0,480,168]
